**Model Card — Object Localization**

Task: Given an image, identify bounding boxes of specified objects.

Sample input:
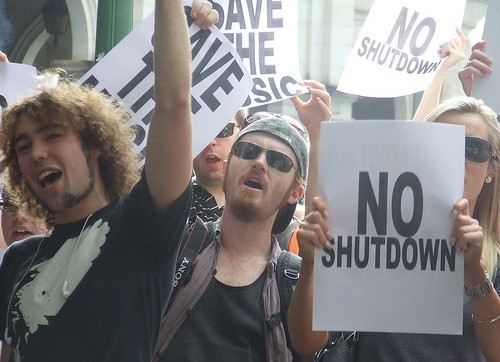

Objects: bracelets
[471,314,500,324]
[463,269,494,299]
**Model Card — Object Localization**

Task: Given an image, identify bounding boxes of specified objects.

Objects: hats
[232,116,308,235]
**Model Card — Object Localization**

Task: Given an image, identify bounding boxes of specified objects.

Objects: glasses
[465,136,493,162]
[216,123,241,138]
[233,141,299,173]
[0,199,16,210]
[242,110,308,136]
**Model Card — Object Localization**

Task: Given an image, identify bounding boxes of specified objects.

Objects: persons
[0,0,500,362]
